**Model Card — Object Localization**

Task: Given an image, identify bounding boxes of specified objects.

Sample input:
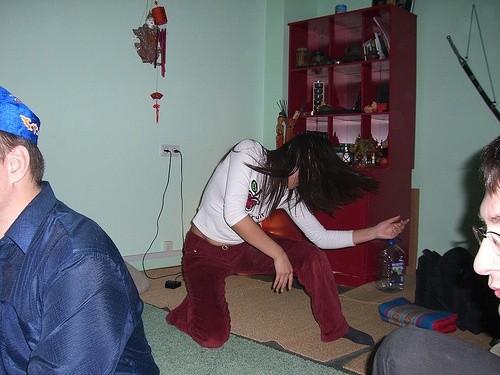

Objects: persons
[166,131,410,348]
[370,136,500,375]
[0,87,161,375]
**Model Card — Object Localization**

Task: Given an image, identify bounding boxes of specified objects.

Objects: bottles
[375,239,406,292]
[312,50,326,65]
[295,48,308,66]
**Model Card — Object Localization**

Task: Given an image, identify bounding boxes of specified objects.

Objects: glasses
[472,226,500,255]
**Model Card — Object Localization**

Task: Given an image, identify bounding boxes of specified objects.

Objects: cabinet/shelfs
[262,3,417,290]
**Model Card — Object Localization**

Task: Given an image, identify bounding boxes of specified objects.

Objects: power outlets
[160,144,181,158]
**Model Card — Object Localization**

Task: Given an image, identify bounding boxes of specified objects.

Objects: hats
[0,86,40,144]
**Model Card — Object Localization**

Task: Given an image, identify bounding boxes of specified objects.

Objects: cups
[335,4,347,13]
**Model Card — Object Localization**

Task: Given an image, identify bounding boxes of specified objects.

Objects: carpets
[136,264,493,375]
